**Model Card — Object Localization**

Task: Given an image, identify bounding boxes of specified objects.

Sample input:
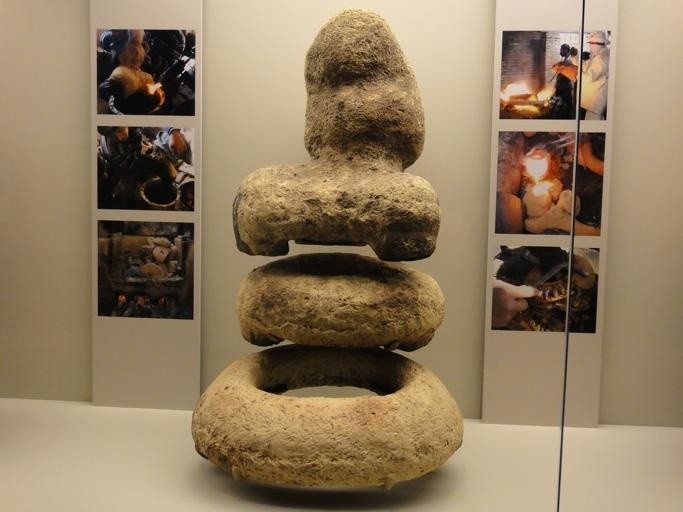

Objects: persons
[491,278,538,327]
[566,133,604,228]
[552,31,610,120]
[522,132,560,153]
[97,127,186,168]
